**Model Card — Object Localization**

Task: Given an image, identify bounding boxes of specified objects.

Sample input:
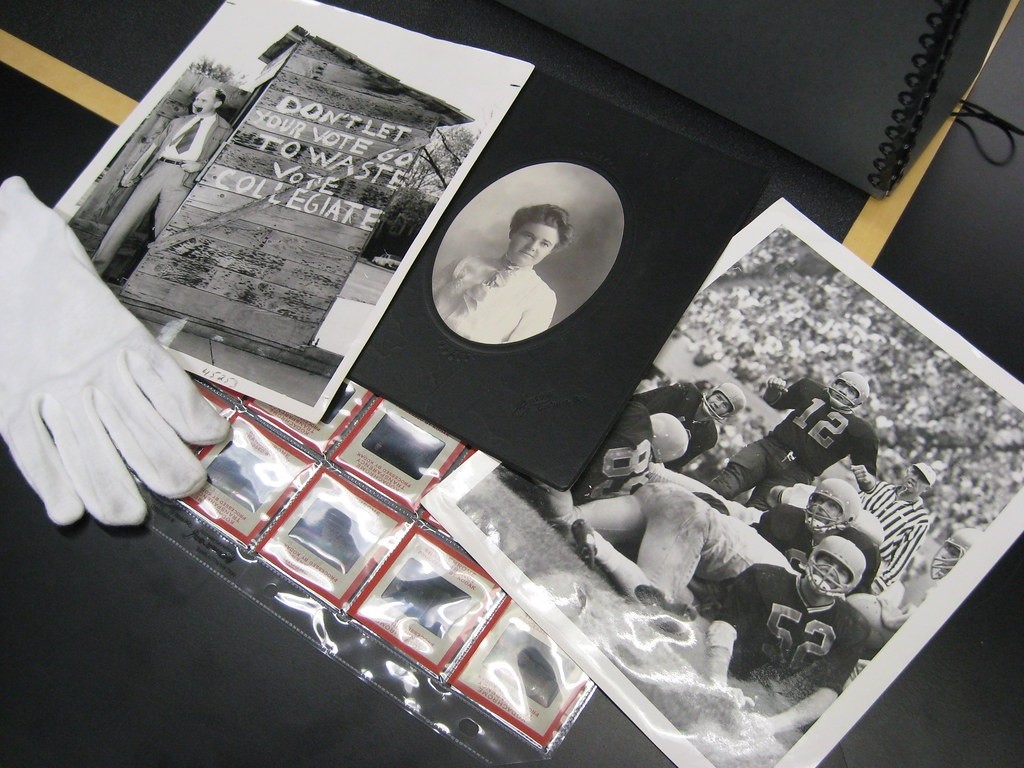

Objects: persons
[431,204,575,348]
[491,370,986,738]
[674,226,1023,548]
[90,87,234,284]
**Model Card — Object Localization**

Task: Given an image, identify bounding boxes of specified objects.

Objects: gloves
[0,175,230,526]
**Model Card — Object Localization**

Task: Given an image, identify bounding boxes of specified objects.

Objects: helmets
[931,528,984,580]
[706,383,745,418]
[804,478,862,538]
[826,371,869,412]
[804,535,867,601]
[650,412,690,465]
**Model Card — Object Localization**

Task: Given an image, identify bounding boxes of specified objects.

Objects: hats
[912,462,935,486]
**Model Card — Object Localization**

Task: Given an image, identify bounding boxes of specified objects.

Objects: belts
[159,156,185,165]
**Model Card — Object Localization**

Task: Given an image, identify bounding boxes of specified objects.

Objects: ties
[175,118,204,155]
